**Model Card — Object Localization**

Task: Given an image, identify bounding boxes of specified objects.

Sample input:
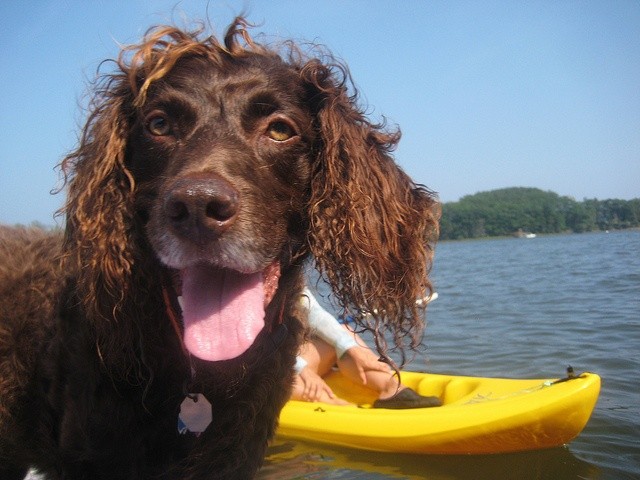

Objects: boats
[271,367,603,456]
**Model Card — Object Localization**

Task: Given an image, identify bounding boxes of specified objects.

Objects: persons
[286,277,442,408]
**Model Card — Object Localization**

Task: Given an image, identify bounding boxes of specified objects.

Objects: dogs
[0,1,443,480]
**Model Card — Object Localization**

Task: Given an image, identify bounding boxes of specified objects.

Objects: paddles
[336,293,438,324]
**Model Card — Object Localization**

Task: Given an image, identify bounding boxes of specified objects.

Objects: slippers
[374,387,442,409]
